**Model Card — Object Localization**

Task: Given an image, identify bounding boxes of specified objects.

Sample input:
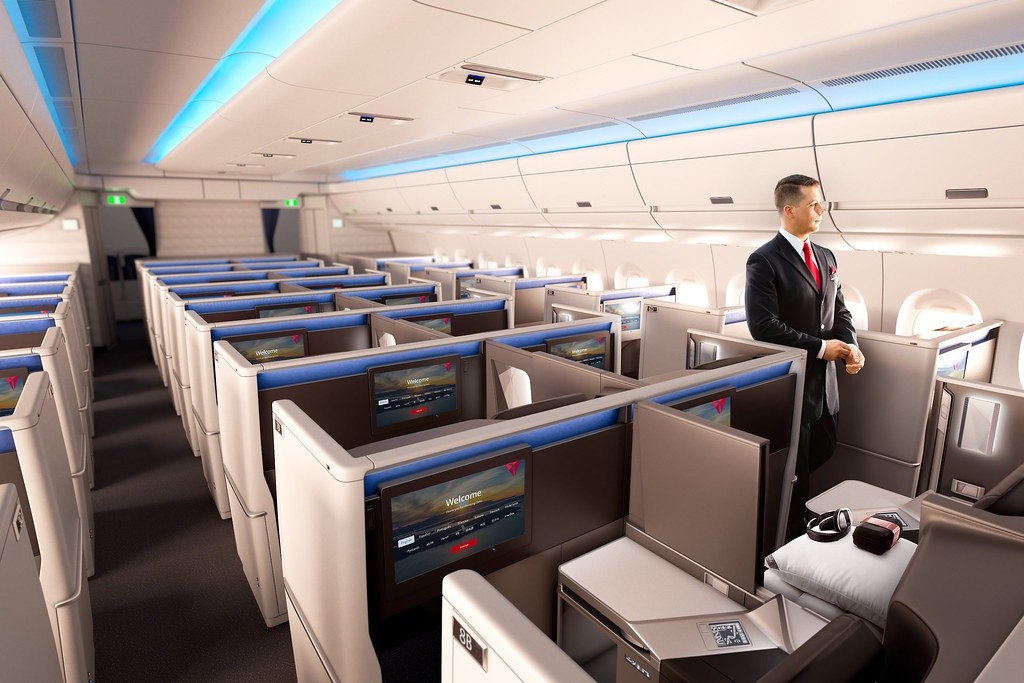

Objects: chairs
[755,598,940,683]
[971,463,1024,517]
[490,391,592,421]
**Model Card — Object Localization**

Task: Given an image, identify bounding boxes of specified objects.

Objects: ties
[803,242,822,293]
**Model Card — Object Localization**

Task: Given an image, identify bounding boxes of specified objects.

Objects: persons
[744,174,866,540]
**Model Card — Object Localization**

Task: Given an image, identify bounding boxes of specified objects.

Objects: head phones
[807,508,853,543]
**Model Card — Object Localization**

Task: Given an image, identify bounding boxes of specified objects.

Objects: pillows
[763,524,920,629]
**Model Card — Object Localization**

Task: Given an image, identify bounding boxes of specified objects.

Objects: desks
[805,479,912,526]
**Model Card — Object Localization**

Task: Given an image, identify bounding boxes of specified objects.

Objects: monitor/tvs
[603,297,643,333]
[544,330,611,372]
[936,343,971,380]
[178,270,481,365]
[377,443,531,599]
[0,302,57,454]
[367,353,462,436]
[665,386,736,429]
